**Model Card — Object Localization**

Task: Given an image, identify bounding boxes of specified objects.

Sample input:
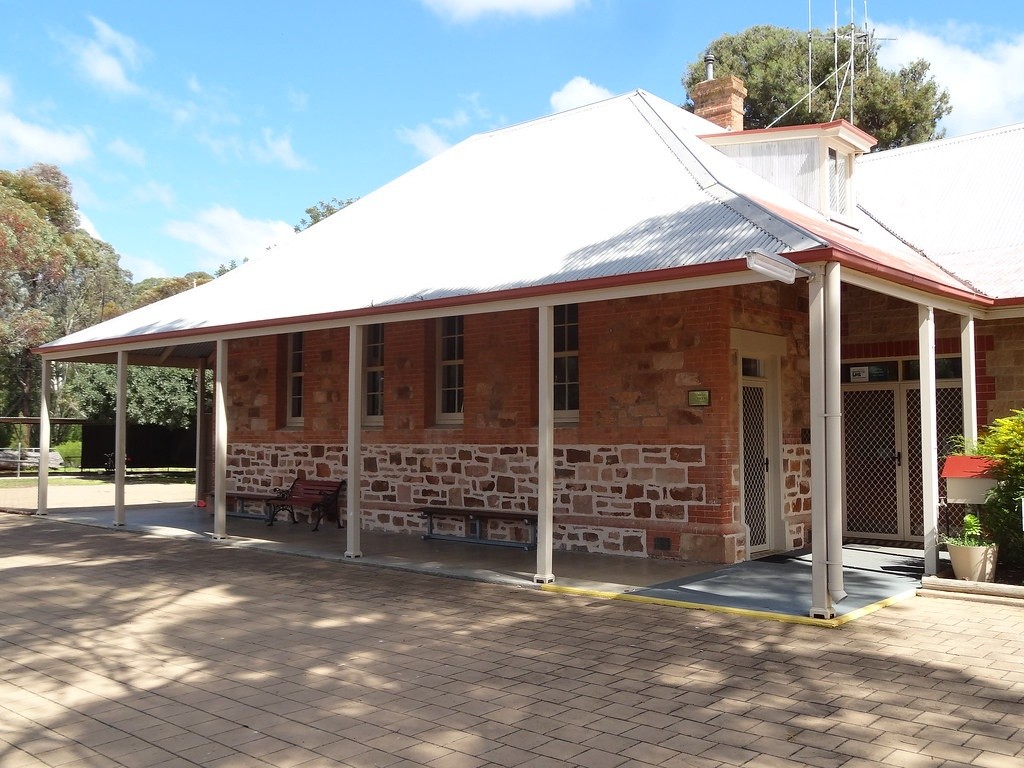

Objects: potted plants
[947,515,999,583]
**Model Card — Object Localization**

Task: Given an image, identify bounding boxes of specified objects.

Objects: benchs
[265,478,345,531]
[410,505,537,550]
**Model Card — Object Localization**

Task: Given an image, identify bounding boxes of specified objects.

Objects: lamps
[745,248,816,285]
